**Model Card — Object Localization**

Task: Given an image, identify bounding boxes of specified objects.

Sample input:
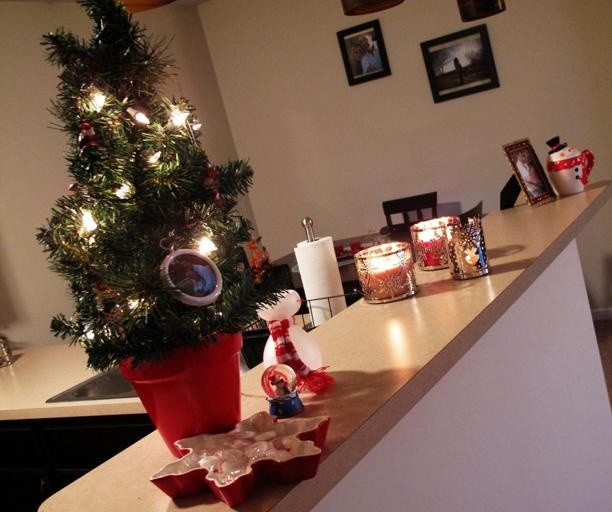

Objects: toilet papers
[292,236,348,328]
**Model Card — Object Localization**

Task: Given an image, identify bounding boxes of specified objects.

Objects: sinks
[44,365,139,403]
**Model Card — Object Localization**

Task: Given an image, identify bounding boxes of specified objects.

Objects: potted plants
[31,0,283,464]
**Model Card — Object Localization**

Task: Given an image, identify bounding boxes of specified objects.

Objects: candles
[353,215,460,305]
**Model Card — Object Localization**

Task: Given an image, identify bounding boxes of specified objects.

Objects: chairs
[383,190,437,224]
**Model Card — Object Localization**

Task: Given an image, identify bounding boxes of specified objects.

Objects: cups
[444,215,490,281]
[409,216,463,272]
[354,240,418,305]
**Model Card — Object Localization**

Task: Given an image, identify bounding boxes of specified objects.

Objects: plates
[333,241,379,260]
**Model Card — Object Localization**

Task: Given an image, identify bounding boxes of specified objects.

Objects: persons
[515,150,544,198]
[349,40,377,77]
[357,34,383,75]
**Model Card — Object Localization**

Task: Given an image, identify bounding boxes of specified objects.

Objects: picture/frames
[335,0,506,105]
[502,137,557,207]
